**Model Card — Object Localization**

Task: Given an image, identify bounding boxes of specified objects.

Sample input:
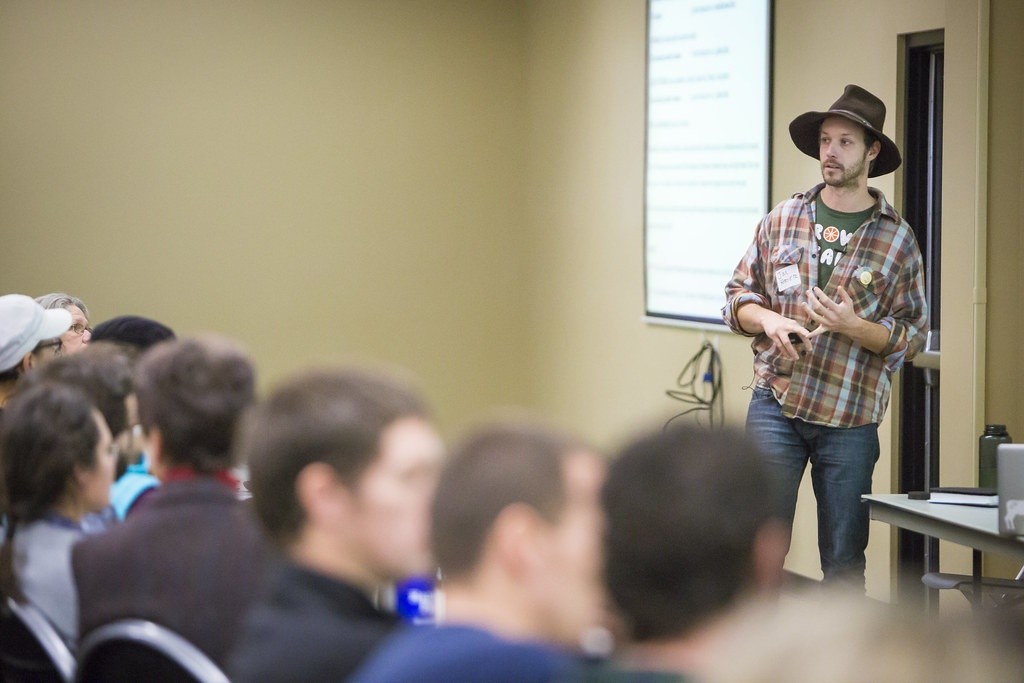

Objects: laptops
[997,443,1024,536]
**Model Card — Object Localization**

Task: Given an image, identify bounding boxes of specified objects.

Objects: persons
[722,83,930,611]
[0,291,1007,683]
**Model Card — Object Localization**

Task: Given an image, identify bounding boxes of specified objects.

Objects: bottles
[979,423,1012,490]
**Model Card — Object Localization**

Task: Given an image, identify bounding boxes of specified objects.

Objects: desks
[861,494,1024,561]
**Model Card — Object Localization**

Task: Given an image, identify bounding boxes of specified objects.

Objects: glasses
[130,423,143,439]
[41,338,62,351]
[71,323,91,335]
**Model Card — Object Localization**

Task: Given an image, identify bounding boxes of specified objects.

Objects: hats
[789,84,902,178]
[0,294,72,374]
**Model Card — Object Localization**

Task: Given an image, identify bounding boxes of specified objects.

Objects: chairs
[0,593,76,683]
[76,620,228,683]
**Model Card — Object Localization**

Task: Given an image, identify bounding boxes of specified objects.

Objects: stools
[922,572,1023,615]
